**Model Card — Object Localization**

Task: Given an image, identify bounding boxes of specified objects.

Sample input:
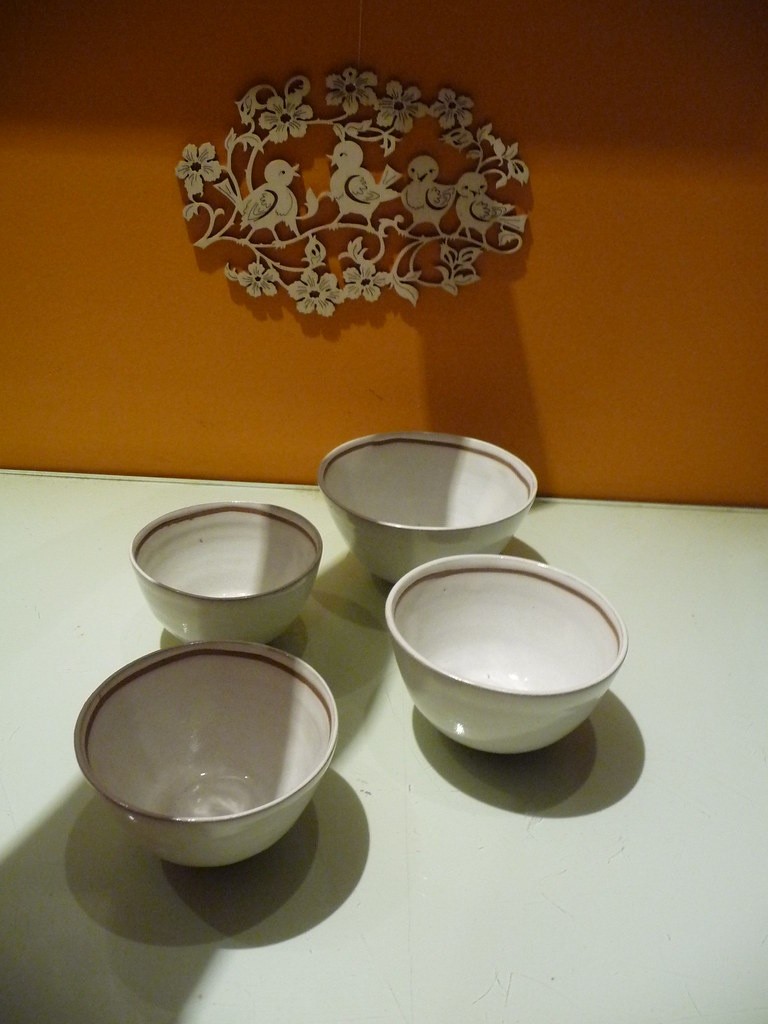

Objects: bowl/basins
[129,503,323,649]
[315,429,539,585]
[73,643,341,869]
[383,552,627,757]
